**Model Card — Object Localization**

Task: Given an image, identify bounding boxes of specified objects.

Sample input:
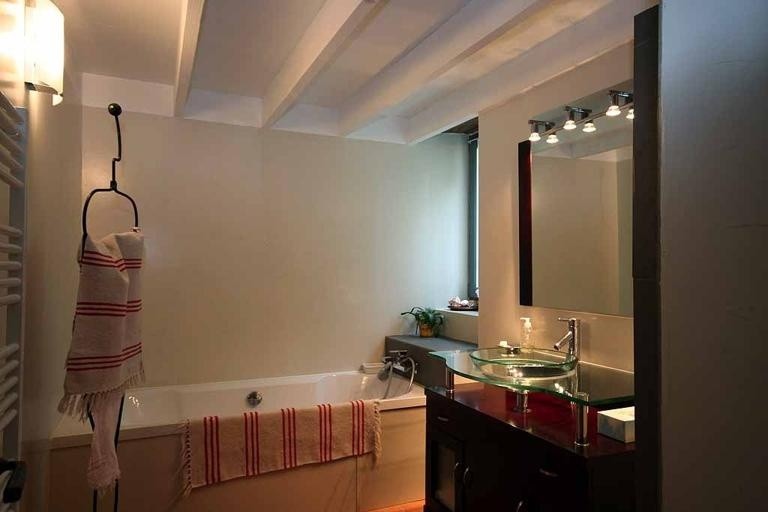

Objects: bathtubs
[51,368,429,450]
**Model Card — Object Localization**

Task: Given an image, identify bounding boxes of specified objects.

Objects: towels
[176,398,380,501]
[57,230,147,499]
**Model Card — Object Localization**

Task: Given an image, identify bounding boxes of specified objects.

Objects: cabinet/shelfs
[423,381,635,512]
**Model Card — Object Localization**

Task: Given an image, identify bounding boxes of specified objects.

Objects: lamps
[606,89,633,117]
[583,120,596,133]
[25,0,66,95]
[528,119,555,142]
[546,132,559,144]
[626,106,634,120]
[563,106,592,130]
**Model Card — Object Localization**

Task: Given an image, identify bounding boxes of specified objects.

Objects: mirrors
[518,101,634,320]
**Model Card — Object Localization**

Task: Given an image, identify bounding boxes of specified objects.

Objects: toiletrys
[519,317,534,353]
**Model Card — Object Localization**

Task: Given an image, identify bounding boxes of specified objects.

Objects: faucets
[553,316,579,361]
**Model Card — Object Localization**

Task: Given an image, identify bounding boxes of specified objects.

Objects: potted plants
[401,306,445,338]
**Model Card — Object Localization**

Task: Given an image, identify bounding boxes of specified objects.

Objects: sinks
[469,346,578,381]
